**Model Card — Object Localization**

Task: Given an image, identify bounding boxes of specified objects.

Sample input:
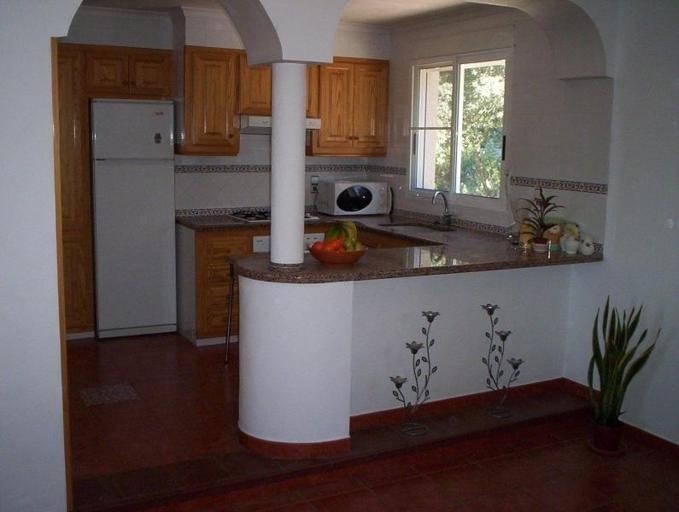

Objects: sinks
[377,221,453,233]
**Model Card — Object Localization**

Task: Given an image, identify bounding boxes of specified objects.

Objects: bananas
[325,220,357,240]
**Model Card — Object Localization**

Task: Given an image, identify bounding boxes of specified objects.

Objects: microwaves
[317,179,393,216]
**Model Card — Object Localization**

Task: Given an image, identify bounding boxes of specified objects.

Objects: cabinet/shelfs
[175,45,244,156]
[304,227,441,253]
[87,46,172,100]
[306,56,388,158]
[53,44,94,338]
[239,66,273,116]
[176,224,272,344]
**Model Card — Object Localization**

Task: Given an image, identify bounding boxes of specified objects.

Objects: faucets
[432,190,452,224]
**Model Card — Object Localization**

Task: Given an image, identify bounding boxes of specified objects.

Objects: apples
[312,238,362,253]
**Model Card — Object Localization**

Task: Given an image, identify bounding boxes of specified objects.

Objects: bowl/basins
[306,241,368,264]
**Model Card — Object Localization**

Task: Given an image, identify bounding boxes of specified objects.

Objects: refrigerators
[90,97,178,338]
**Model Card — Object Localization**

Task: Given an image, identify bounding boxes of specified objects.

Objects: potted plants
[582,297,660,456]
[515,186,566,253]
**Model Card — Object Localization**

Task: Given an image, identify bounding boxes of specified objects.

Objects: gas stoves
[229,209,320,223]
